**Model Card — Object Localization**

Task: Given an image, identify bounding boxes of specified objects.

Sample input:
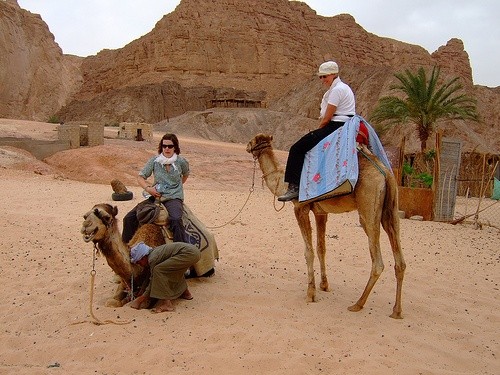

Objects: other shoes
[184,267,191,276]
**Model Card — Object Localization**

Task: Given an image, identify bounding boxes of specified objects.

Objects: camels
[80,204,165,309]
[246,133,407,319]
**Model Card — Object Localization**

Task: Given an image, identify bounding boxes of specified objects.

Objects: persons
[121,133,190,243]
[131,242,202,313]
[278,61,355,201]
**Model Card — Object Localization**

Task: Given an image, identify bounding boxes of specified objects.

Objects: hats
[319,61,339,76]
[131,241,152,264]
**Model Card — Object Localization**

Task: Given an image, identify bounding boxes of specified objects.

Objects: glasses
[162,144,174,149]
[319,75,328,79]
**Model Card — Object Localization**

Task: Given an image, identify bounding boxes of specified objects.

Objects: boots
[278,183,299,202]
